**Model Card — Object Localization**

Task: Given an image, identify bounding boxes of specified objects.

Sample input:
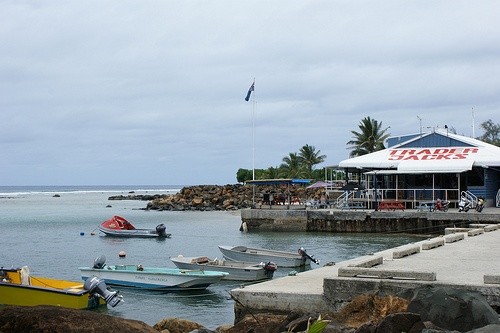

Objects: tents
[246,179,311,205]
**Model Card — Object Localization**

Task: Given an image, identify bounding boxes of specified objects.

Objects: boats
[77,255,230,295]
[97,215,172,238]
[168,254,278,282]
[217,244,320,269]
[0,265,126,311]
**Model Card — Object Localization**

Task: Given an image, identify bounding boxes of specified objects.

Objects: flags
[244,82,254,101]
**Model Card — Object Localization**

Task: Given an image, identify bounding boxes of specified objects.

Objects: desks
[343,202,364,211]
[380,202,403,211]
[419,202,434,212]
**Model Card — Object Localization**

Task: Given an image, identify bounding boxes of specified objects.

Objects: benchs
[414,206,436,209]
[342,205,367,208]
[379,205,405,209]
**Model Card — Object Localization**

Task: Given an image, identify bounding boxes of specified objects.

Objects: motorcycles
[430,198,450,212]
[458,195,471,212]
[475,195,486,212]
